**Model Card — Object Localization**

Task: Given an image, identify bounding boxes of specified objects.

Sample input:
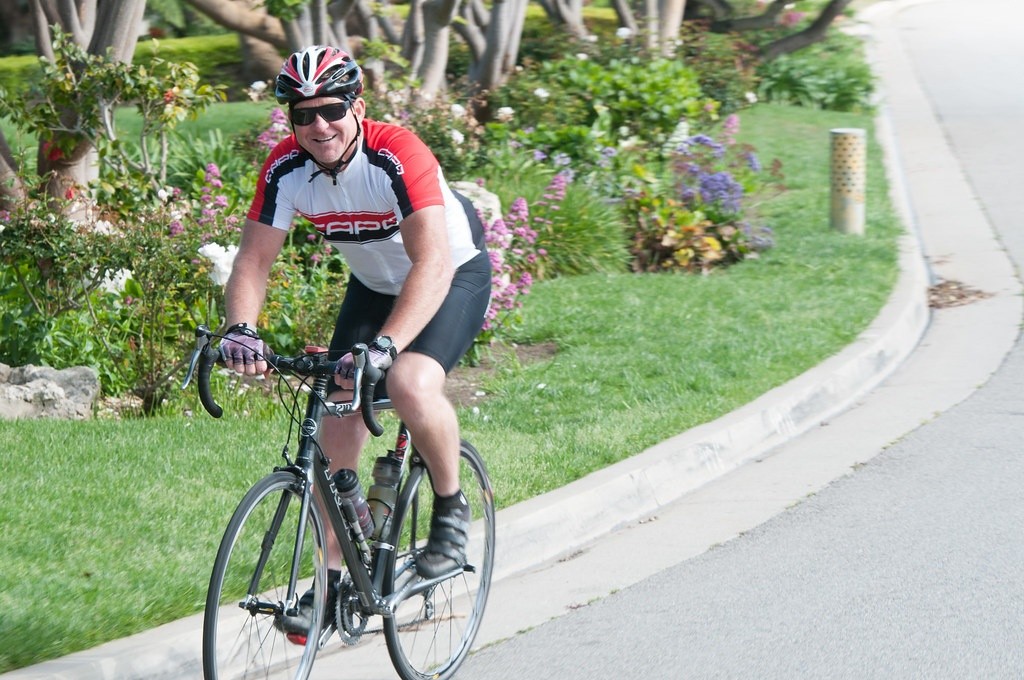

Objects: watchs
[369,334,399,361]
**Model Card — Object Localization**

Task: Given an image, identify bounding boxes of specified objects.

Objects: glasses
[289,99,355,126]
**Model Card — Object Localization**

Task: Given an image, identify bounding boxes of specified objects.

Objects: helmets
[275,45,364,105]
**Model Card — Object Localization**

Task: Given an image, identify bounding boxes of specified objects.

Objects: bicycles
[180,326,498,680]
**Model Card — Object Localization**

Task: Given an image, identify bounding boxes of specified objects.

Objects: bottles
[367,449,402,542]
[332,468,375,538]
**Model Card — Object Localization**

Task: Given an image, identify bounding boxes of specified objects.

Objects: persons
[216,44,493,641]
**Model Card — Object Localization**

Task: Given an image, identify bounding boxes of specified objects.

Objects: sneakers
[416,503,471,579]
[273,590,350,635]
